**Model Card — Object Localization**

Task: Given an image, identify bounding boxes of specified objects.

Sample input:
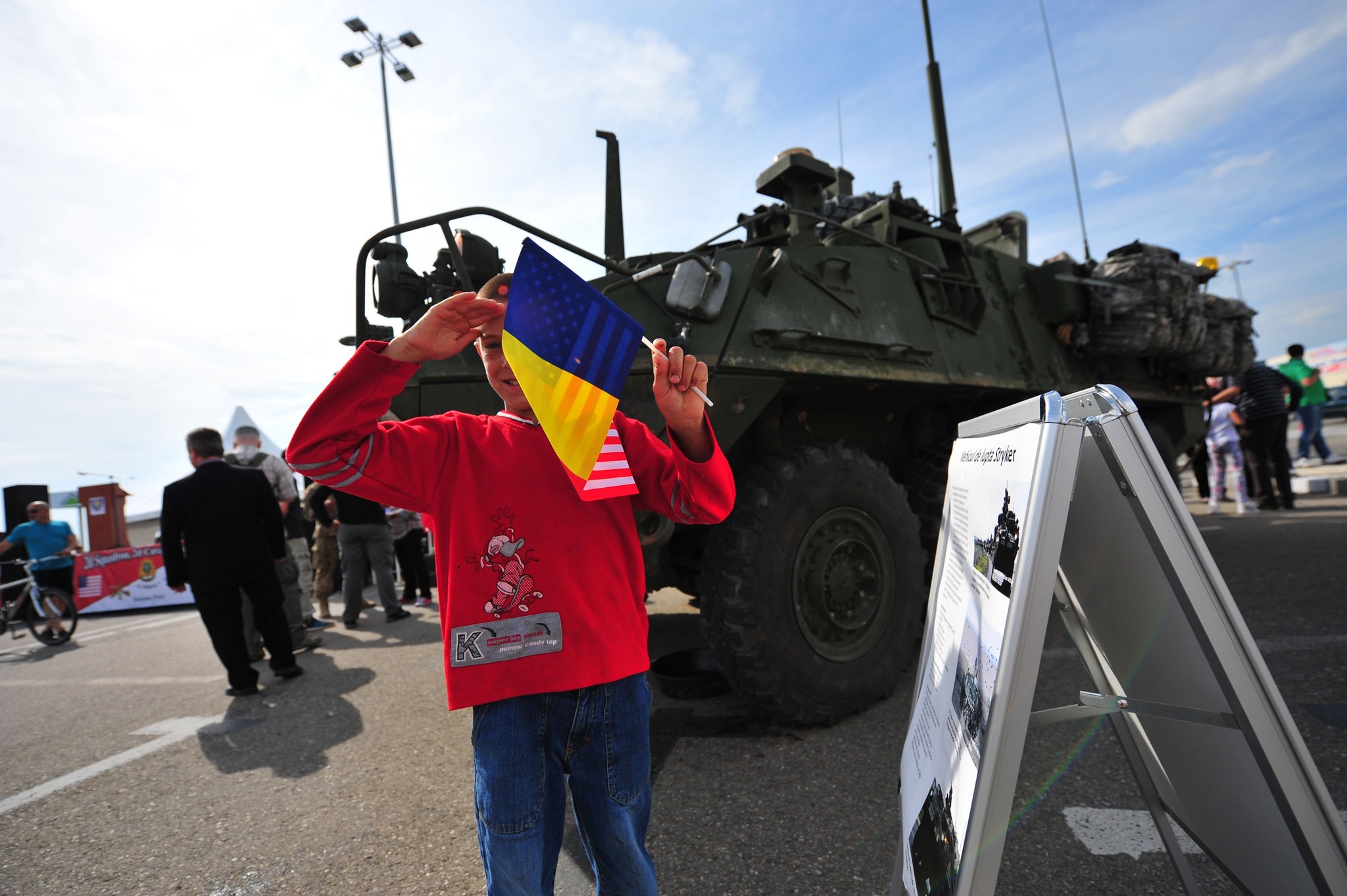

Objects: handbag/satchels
[412,531,429,556]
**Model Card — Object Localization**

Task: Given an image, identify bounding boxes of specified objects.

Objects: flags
[501,242,645,502]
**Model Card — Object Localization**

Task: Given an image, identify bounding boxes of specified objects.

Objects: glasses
[26,509,38,514]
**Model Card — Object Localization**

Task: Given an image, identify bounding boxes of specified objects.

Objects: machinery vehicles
[336,2,1258,733]
[912,777,960,896]
[959,656,983,740]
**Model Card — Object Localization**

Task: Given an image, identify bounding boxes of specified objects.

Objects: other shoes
[387,608,410,621]
[274,664,303,679]
[58,630,71,641]
[40,629,55,639]
[225,685,258,696]
[246,647,265,662]
[344,620,356,628]
[1208,498,1220,515]
[361,598,376,608]
[318,598,330,617]
[1292,457,1307,468]
[1237,502,1260,514]
[1324,454,1336,464]
[1257,502,1276,510]
[303,618,335,631]
[415,597,431,606]
[1280,501,1293,509]
[294,635,322,650]
[398,597,408,602]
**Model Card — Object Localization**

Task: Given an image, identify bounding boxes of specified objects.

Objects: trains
[974,480,1021,595]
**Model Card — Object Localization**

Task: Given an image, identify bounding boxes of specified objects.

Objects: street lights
[339,14,424,244]
[1215,259,1255,301]
[78,471,121,548]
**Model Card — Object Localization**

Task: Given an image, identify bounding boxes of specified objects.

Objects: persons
[302,477,432,629]
[1279,344,1347,466]
[223,425,322,659]
[160,428,302,695]
[0,502,78,640]
[1188,359,1304,514]
[286,273,735,896]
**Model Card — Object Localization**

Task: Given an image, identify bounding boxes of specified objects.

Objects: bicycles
[0,551,79,646]
